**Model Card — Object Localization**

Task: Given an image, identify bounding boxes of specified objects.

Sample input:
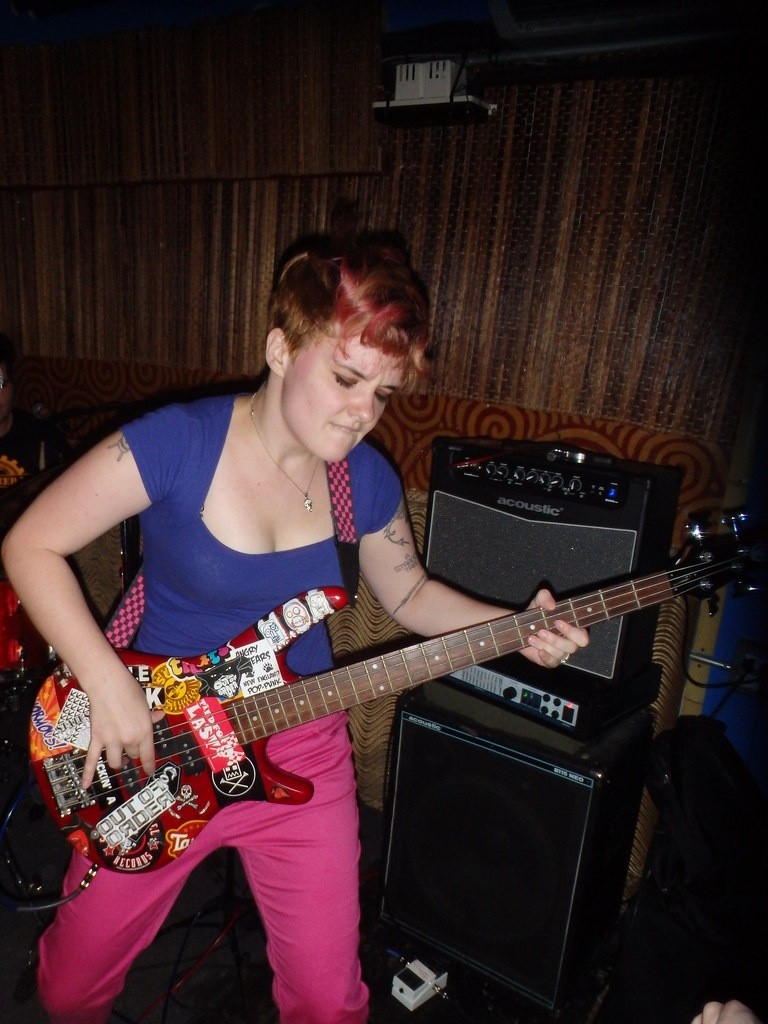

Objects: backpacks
[609,715,766,1023]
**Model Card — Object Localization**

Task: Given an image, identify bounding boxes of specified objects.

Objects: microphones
[32,403,80,440]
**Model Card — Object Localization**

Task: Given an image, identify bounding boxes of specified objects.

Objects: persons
[0,333,53,489]
[0,232,590,1024]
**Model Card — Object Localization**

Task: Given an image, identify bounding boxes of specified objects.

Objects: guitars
[27,504,767,877]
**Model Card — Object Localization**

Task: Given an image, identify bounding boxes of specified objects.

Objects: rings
[562,653,569,664]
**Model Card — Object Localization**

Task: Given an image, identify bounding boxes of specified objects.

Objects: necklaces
[250,393,317,513]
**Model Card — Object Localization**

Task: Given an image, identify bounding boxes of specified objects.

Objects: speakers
[374,681,655,1019]
[420,435,684,741]
